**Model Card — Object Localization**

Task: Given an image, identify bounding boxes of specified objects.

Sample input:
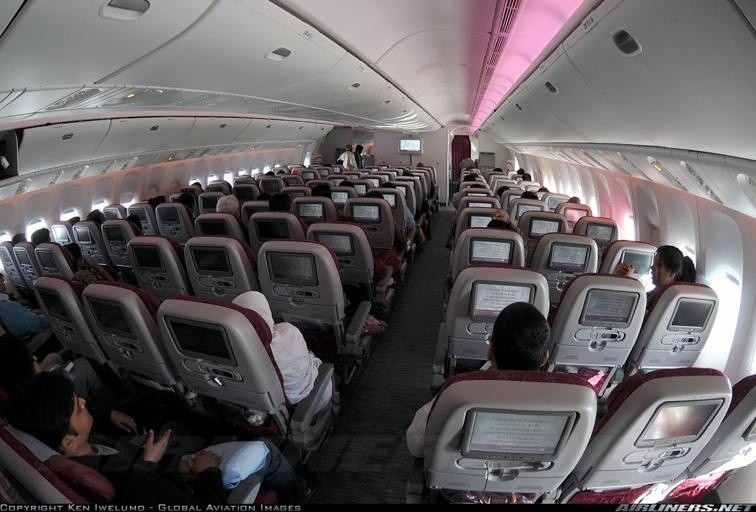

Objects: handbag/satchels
[360,315,388,335]
[180,442,270,486]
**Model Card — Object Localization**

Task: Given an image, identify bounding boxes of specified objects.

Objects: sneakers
[296,479,319,501]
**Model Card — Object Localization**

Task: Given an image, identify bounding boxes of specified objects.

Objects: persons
[610,246,696,322]
[1,145,425,512]
[463,165,582,256]
[406,303,549,460]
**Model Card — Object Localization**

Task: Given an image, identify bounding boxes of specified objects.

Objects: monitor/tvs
[0,163,756,462]
[397,137,423,156]
